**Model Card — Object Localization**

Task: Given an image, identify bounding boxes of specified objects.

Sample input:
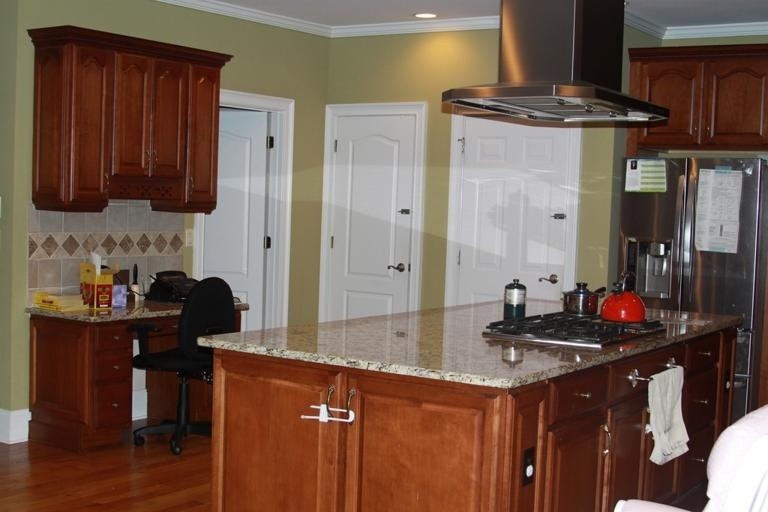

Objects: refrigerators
[618,153,768,424]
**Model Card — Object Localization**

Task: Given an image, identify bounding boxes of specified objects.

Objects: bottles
[505,279,526,320]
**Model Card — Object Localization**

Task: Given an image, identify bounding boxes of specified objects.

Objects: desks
[23,302,249,323]
[198,299,742,390]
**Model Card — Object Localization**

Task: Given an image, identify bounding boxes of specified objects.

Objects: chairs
[133,277,234,455]
[613,402,768,512]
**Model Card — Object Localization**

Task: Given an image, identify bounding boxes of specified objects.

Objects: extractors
[441,0,670,128]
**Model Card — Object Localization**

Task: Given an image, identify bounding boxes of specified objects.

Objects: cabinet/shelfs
[135,310,241,438]
[212,347,506,512]
[28,25,108,212]
[108,32,188,201]
[506,366,607,512]
[607,340,686,512]
[150,47,234,214]
[678,326,738,496]
[627,43,768,157]
[30,315,133,448]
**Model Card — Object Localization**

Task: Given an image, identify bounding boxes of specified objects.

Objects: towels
[649,366,690,465]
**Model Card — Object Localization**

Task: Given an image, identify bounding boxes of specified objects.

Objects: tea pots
[600,272,645,323]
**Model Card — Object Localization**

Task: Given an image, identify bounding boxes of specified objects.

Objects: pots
[565,283,605,314]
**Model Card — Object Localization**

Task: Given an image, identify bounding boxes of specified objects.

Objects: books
[32,293,91,313]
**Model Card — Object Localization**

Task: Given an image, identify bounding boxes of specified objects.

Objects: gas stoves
[485,312,665,348]
